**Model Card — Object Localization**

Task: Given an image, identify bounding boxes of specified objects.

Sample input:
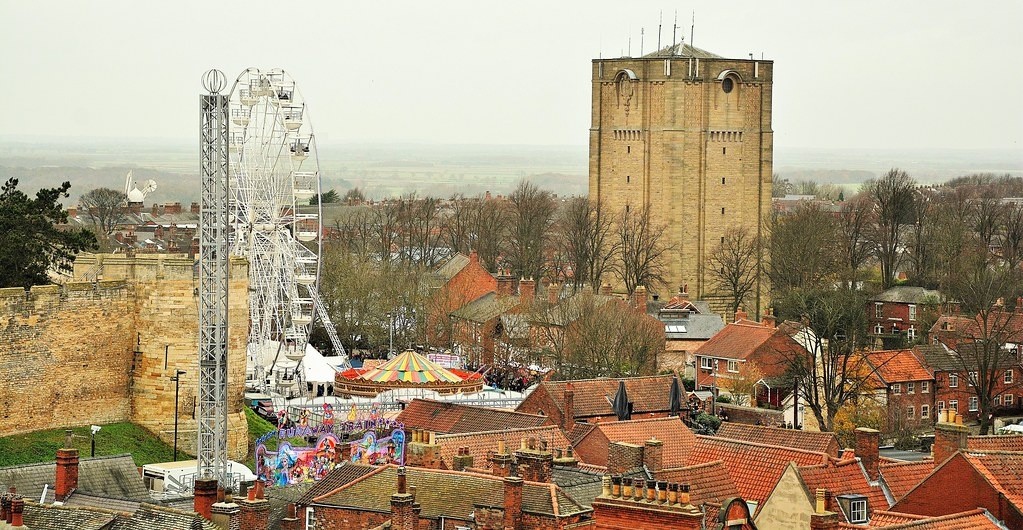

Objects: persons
[719,406,729,422]
[278,93,288,99]
[317,349,337,356]
[694,406,699,416]
[797,423,802,429]
[352,349,391,362]
[767,421,771,426]
[307,382,313,391]
[756,419,761,425]
[317,384,325,396]
[787,421,793,429]
[290,147,295,152]
[302,147,309,152]
[466,360,539,392]
[285,116,290,119]
[327,384,333,395]
[781,420,786,428]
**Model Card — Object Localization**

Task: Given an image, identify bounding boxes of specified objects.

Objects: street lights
[386,311,397,352]
[709,358,719,417]
[90,425,101,459]
[168,368,186,464]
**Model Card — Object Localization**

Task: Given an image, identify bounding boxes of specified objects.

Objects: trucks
[141,458,263,501]
